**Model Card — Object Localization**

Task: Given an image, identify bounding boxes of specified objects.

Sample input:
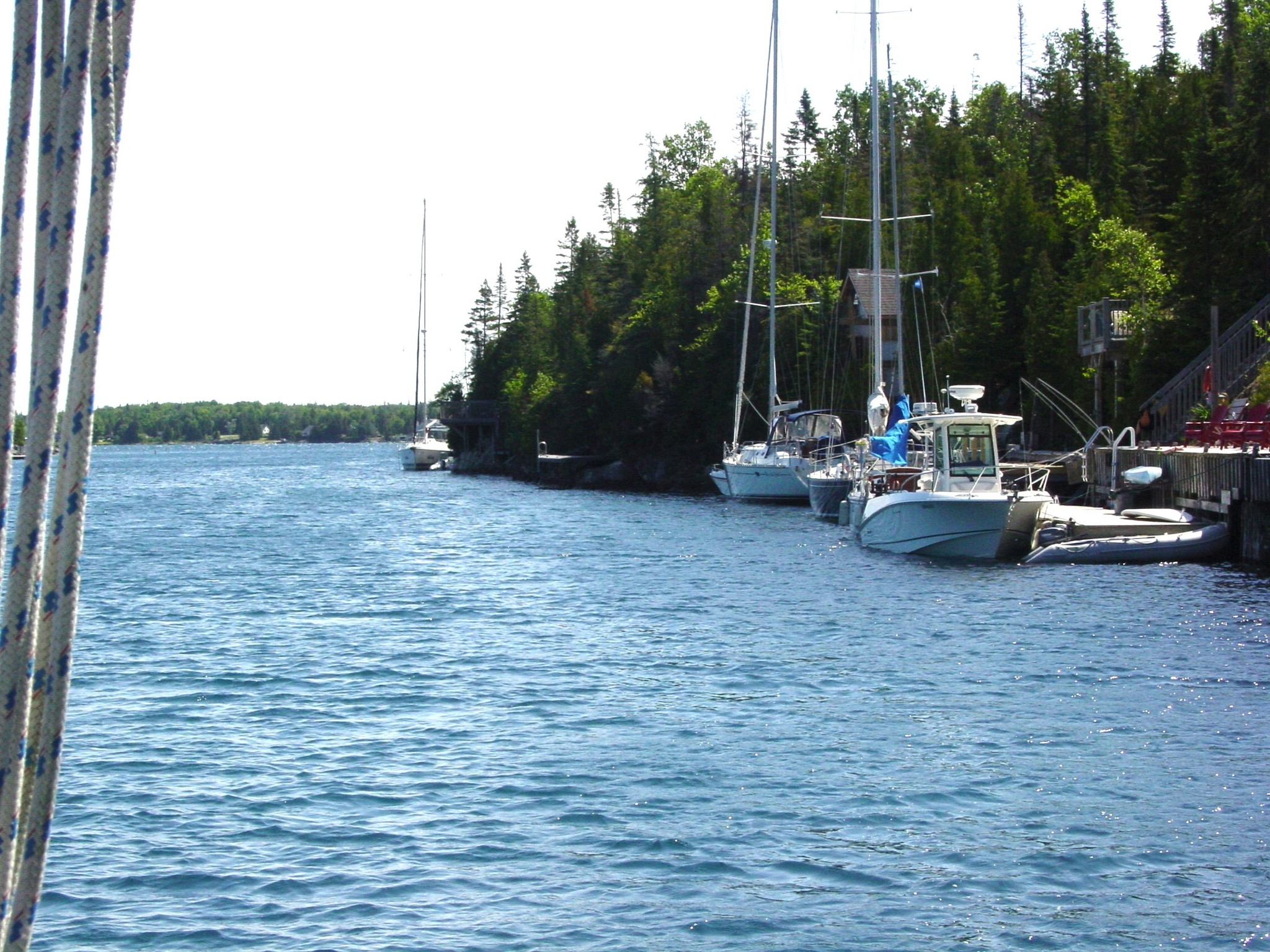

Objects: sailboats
[398,199,454,471]
[707,0,1233,571]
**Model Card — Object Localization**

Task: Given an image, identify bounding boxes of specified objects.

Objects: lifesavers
[842,454,851,472]
[1202,365,1211,392]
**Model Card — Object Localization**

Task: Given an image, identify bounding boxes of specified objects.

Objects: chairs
[1184,401,1270,448]
